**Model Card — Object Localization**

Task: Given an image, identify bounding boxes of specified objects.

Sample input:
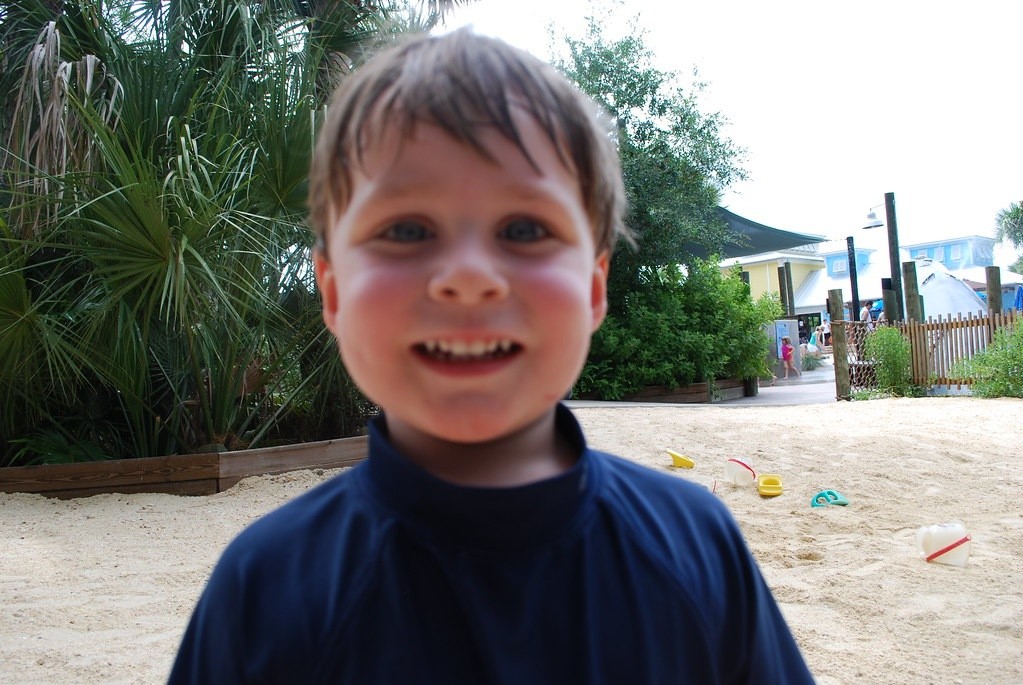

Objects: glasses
[871,304,873,308]
[821,329,824,331]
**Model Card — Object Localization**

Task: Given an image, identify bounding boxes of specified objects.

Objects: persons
[859,300,874,330]
[807,325,825,356]
[819,319,831,346]
[160,28,816,685]
[875,312,885,331]
[780,335,801,381]
